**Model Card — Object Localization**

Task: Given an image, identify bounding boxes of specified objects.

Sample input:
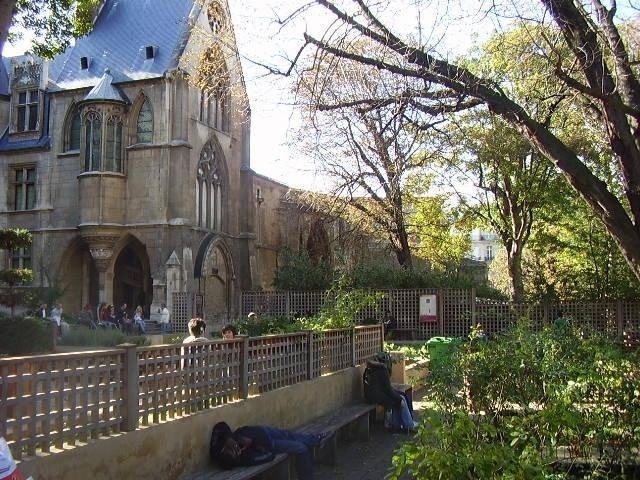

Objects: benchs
[180,382,415,480]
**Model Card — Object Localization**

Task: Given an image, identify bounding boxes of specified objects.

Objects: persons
[379,351,420,428]
[36,298,263,394]
[362,349,419,434]
[382,310,398,338]
[208,418,335,480]
